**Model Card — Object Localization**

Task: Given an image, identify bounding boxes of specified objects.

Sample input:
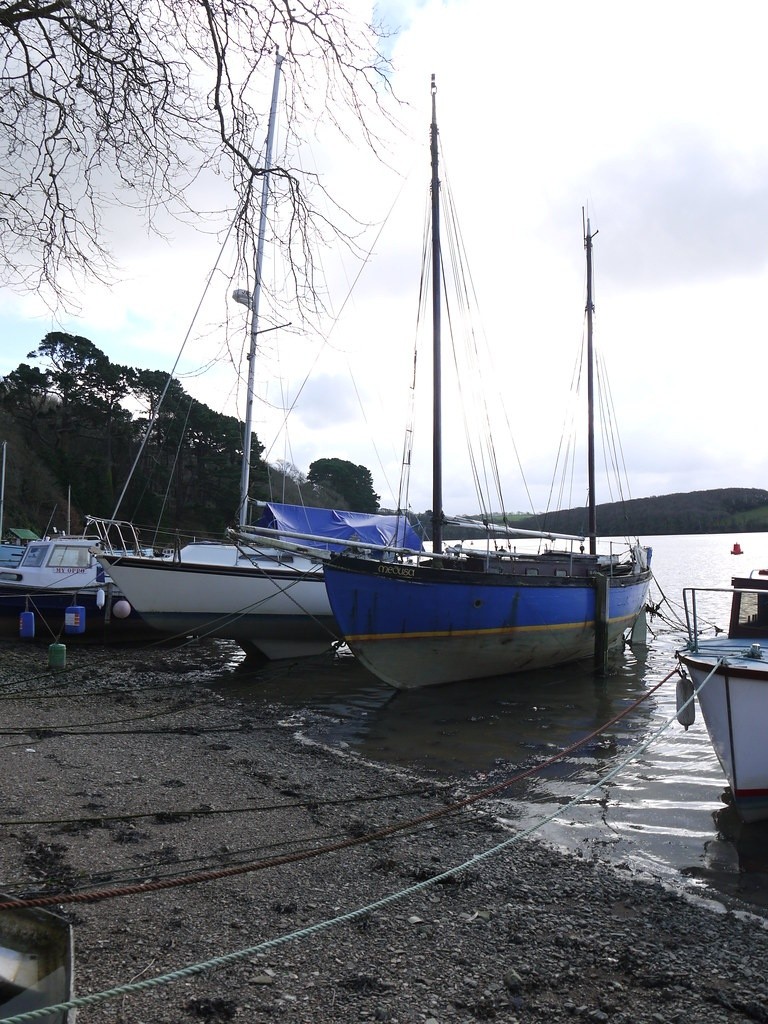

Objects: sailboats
[83,64,424,624]
[221,72,654,696]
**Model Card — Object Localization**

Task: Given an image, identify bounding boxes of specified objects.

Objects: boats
[674,571,767,816]
[0,534,143,632]
